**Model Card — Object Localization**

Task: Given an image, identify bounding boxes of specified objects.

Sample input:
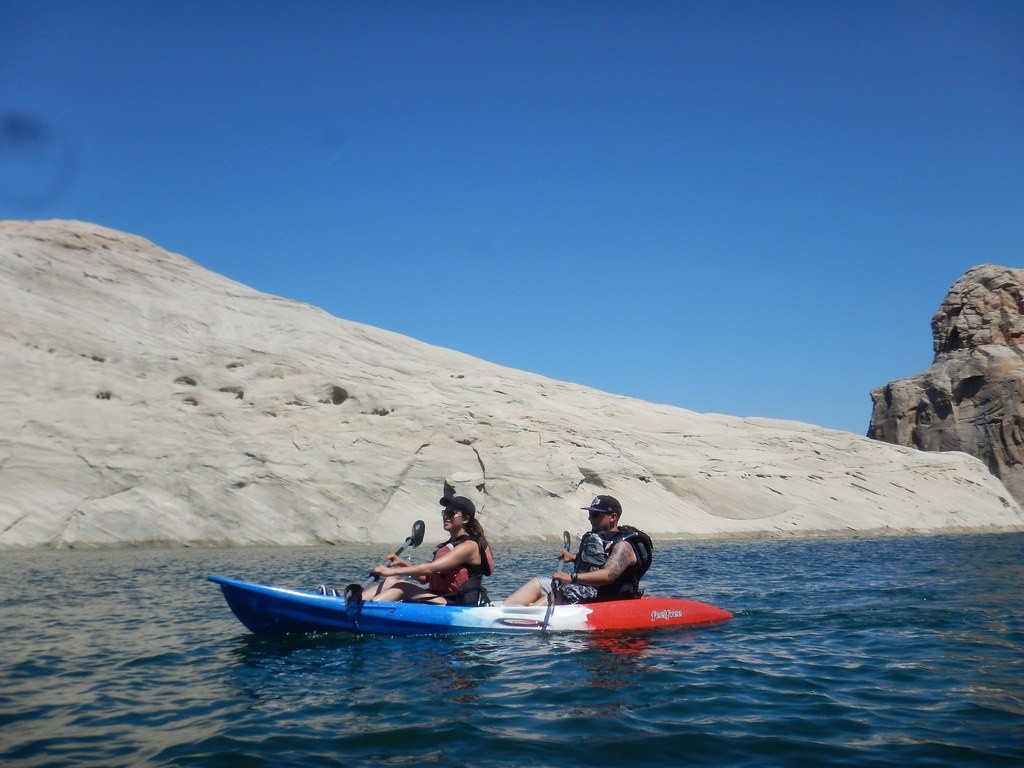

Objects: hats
[580,495,622,517]
[440,496,475,518]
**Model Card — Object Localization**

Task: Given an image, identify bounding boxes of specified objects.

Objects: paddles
[347,520,425,593]
[538,530,570,634]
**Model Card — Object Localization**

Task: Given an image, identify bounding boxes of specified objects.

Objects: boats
[201,574,733,644]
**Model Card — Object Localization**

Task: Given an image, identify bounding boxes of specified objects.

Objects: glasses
[441,510,463,518]
[589,511,617,518]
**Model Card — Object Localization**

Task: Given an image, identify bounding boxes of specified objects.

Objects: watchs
[571,572,578,583]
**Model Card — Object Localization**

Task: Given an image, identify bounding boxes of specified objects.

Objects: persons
[487,496,638,607]
[317,494,496,604]
